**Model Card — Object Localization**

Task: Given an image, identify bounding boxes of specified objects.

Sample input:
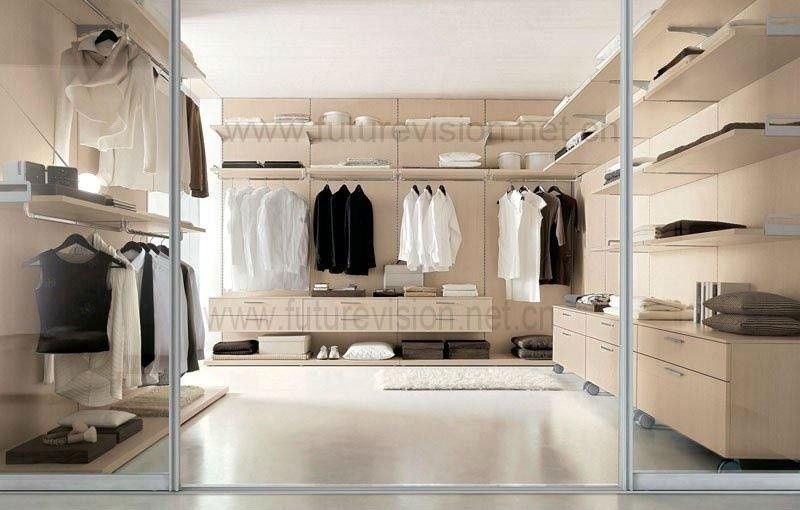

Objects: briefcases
[383,260,424,290]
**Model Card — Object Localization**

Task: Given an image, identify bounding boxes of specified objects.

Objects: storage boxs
[322,110,352,125]
[384,264,423,289]
[521,151,556,172]
[354,115,381,126]
[401,340,444,359]
[259,334,312,355]
[497,151,524,171]
[446,340,490,359]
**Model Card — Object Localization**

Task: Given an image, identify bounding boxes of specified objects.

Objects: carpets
[382,367,561,391]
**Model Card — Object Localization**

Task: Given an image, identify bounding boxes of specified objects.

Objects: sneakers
[329,346,340,360]
[316,345,328,360]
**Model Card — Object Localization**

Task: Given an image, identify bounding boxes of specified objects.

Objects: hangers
[410,177,448,198]
[20,216,127,269]
[153,62,169,83]
[227,176,290,193]
[496,180,564,208]
[321,177,365,199]
[120,229,170,260]
[95,14,120,49]
[86,223,99,251]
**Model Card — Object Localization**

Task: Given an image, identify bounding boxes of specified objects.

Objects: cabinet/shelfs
[303,297,398,332]
[210,299,303,333]
[210,118,575,179]
[397,296,494,332]
[2,0,214,242]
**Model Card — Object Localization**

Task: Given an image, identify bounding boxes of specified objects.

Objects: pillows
[438,159,482,169]
[57,410,136,427]
[510,346,552,359]
[439,151,482,162]
[511,334,552,350]
[341,341,396,360]
